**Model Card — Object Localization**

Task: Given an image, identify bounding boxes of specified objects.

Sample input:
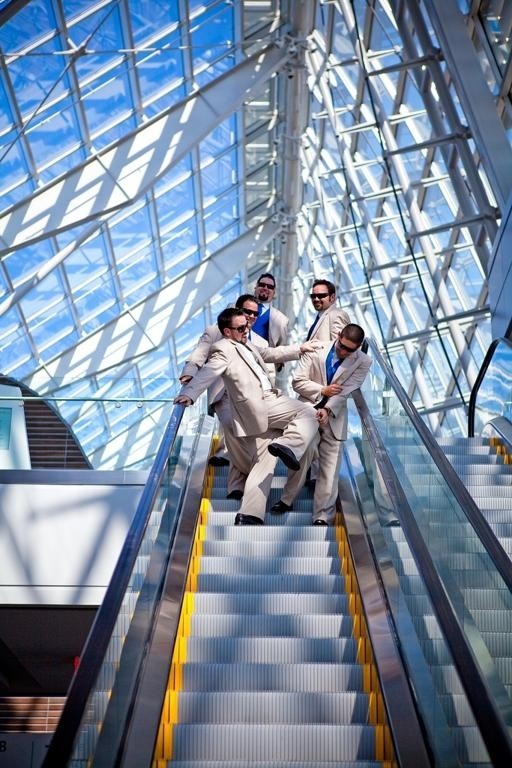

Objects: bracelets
[324,408,334,418]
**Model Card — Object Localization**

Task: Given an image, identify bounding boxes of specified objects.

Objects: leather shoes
[226,489,243,500]
[268,443,300,471]
[234,513,263,524]
[270,501,293,514]
[314,520,329,525]
[210,456,229,466]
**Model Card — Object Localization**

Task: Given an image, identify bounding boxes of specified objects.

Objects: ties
[259,303,264,315]
[307,313,320,340]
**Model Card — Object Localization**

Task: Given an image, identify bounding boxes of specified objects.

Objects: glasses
[258,282,275,289]
[338,339,360,352]
[310,293,329,299]
[241,308,259,316]
[229,321,248,332]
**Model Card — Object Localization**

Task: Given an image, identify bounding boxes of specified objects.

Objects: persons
[271,323,373,525]
[210,273,290,466]
[174,308,320,525]
[304,279,351,491]
[180,294,276,499]
